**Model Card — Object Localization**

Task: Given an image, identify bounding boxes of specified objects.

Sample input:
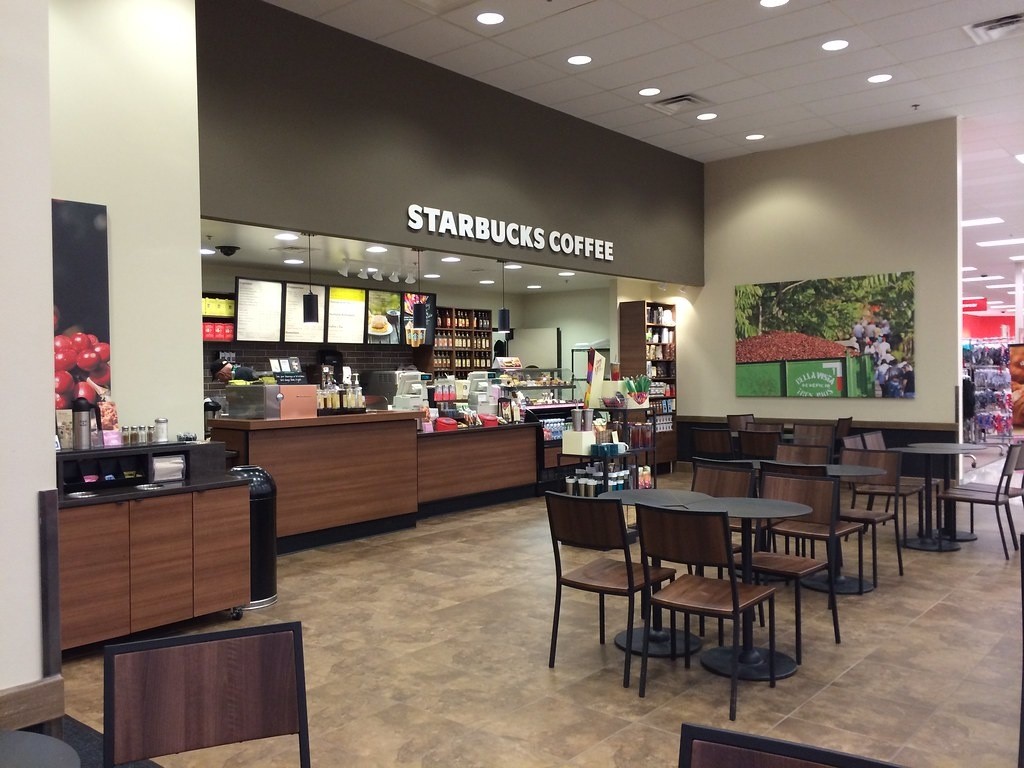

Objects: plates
[368,323,393,336]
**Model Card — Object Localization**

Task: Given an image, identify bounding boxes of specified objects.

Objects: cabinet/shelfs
[415,307,493,380]
[619,300,677,474]
[57,441,253,650]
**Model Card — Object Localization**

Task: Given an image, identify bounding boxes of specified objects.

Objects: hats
[893,359,898,364]
[892,374,899,378]
[901,364,911,369]
[881,359,888,365]
[868,320,874,323]
[901,357,908,361]
[208,358,229,381]
[868,341,873,345]
[882,337,886,341]
[886,349,892,353]
[889,360,895,365]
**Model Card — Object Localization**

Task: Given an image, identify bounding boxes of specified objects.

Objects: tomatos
[54,314,110,409]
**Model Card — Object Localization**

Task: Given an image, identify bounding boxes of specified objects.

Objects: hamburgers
[372,315,388,332]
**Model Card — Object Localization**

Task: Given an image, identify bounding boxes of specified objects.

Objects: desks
[886,448,971,552]
[102,620,312,768]
[729,459,874,595]
[907,443,988,542]
[599,489,813,682]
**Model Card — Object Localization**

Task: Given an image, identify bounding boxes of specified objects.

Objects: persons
[210,359,260,386]
[853,317,915,399]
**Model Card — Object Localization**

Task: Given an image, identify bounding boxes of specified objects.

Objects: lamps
[497,259,510,334]
[301,231,318,323]
[411,246,426,328]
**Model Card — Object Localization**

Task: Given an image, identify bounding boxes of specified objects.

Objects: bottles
[455,310,469,327]
[474,352,491,368]
[454,331,470,348]
[434,331,452,348]
[473,333,490,349]
[455,352,470,368]
[436,310,451,327]
[650,402,663,414]
[434,351,450,368]
[541,418,574,440]
[456,372,469,380]
[316,387,355,410]
[155,419,169,442]
[612,421,652,449]
[474,312,489,330]
[122,425,154,445]
[435,372,450,378]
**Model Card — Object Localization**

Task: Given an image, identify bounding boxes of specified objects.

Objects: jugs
[72,397,102,450]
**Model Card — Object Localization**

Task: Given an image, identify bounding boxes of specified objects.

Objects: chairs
[693,414,1024,588]
[543,491,677,688]
[635,503,776,721]
[685,456,865,644]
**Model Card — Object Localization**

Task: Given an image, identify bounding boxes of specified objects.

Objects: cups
[566,462,631,499]
[571,409,583,431]
[404,328,426,347]
[583,408,594,431]
[610,362,620,381]
